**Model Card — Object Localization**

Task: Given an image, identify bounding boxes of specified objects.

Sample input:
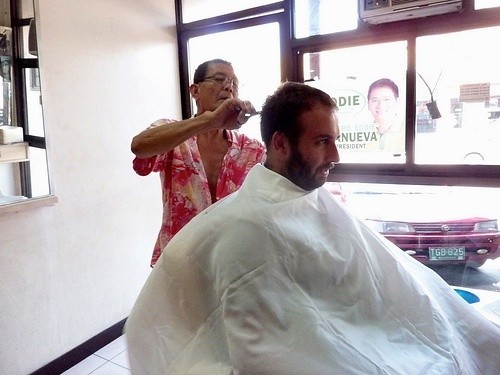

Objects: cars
[341,180,500,274]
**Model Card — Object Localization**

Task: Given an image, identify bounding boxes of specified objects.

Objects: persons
[125,82,499,375]
[130,58,267,268]
[367,80,399,127]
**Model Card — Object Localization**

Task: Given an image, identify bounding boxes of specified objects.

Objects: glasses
[196,75,239,88]
